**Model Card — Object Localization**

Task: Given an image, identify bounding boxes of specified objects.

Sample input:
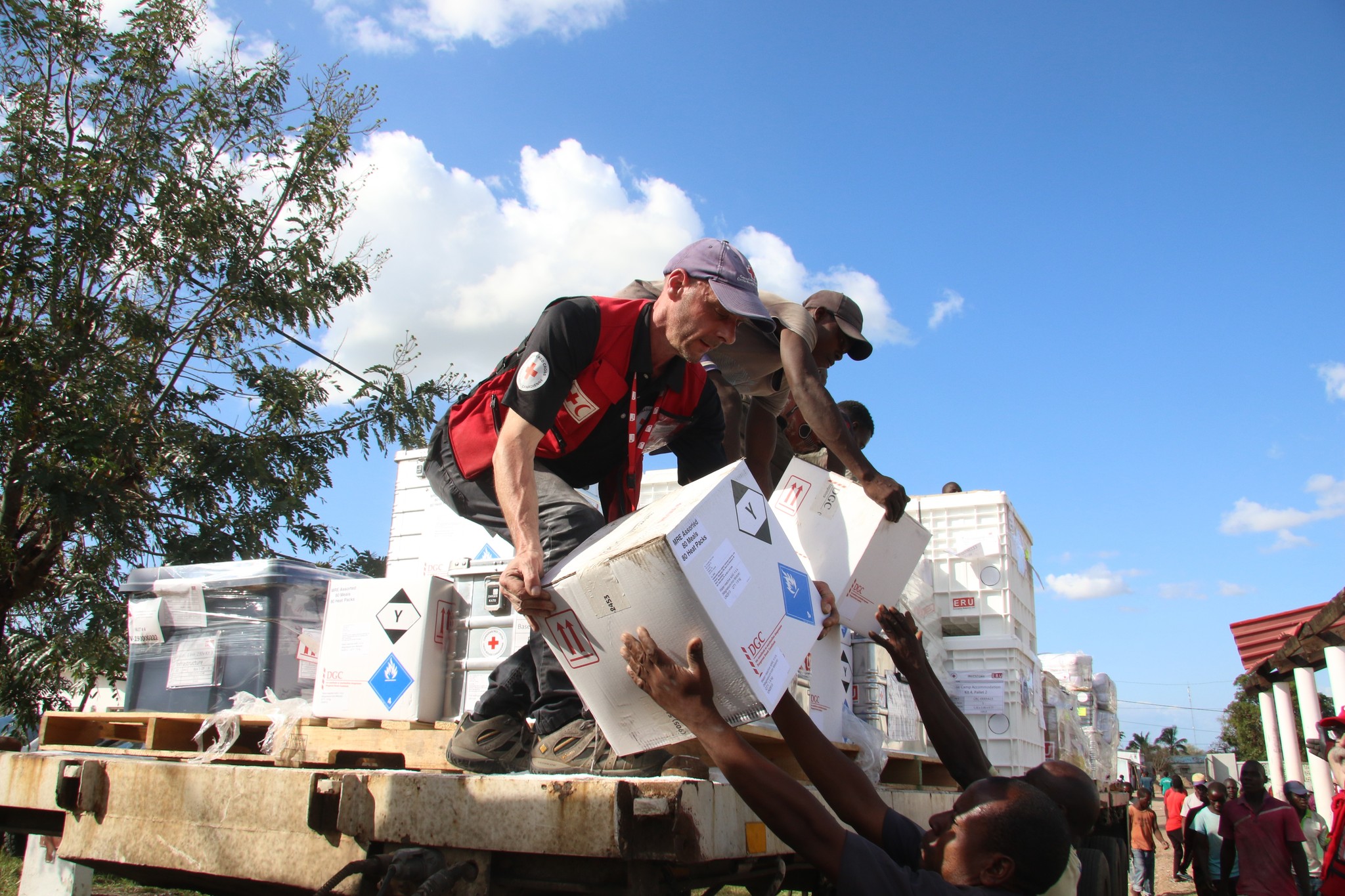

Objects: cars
[0,713,43,753]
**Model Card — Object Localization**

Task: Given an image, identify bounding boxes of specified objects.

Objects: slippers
[1176,872,1194,880]
[1171,878,1190,883]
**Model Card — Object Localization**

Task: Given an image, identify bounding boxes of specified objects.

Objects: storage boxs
[311,573,455,722]
[634,467,685,512]
[524,455,835,760]
[383,447,519,582]
[749,489,1101,778]
[118,557,375,715]
[441,556,531,723]
[767,453,934,641]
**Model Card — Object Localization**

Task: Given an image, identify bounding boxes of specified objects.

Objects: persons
[1159,760,1331,896]
[1305,706,1345,896]
[620,604,1111,896]
[1128,787,1169,896]
[423,238,840,779]
[609,279,910,523]
[942,482,962,494]
[1119,763,1157,809]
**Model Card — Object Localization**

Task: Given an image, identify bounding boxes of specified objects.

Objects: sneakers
[445,713,538,775]
[528,712,673,776]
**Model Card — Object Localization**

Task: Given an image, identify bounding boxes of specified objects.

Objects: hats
[1319,705,1345,728]
[802,289,873,362]
[1283,780,1314,796]
[1191,773,1206,787]
[662,239,777,334]
